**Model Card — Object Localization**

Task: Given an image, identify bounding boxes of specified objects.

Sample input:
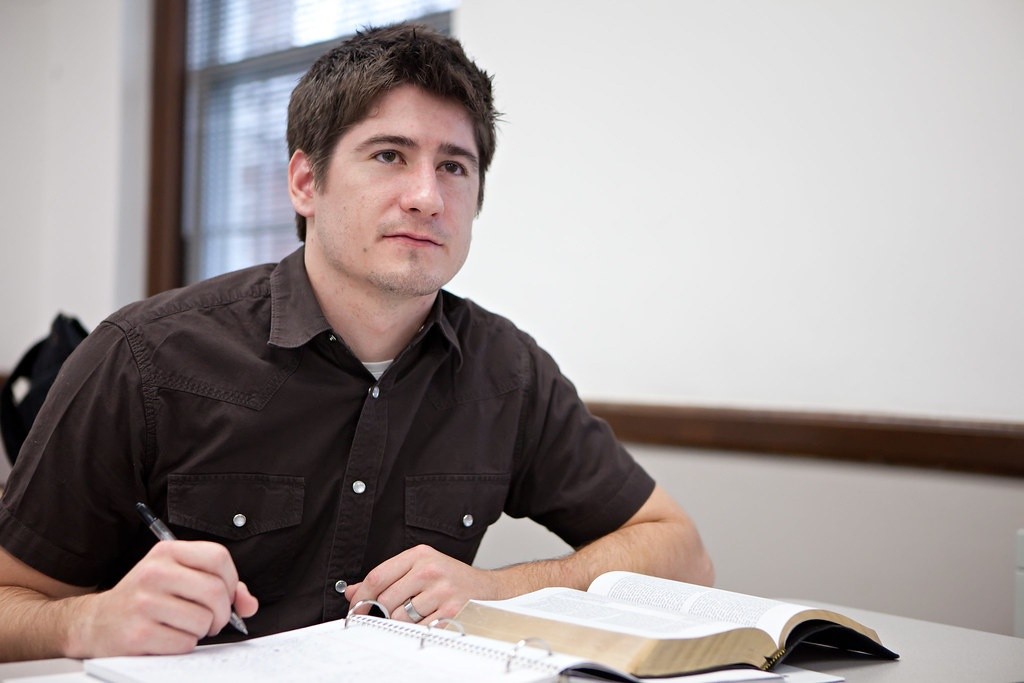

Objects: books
[445,570,899,677]
[80,601,846,682]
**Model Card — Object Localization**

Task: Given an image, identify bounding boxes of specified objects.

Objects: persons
[0,22,713,665]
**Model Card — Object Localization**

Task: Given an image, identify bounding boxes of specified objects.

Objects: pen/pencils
[136,502,250,635]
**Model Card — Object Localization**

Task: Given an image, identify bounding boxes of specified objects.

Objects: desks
[0,598,1024,683]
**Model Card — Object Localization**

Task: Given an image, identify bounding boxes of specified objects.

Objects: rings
[404,597,424,623]
